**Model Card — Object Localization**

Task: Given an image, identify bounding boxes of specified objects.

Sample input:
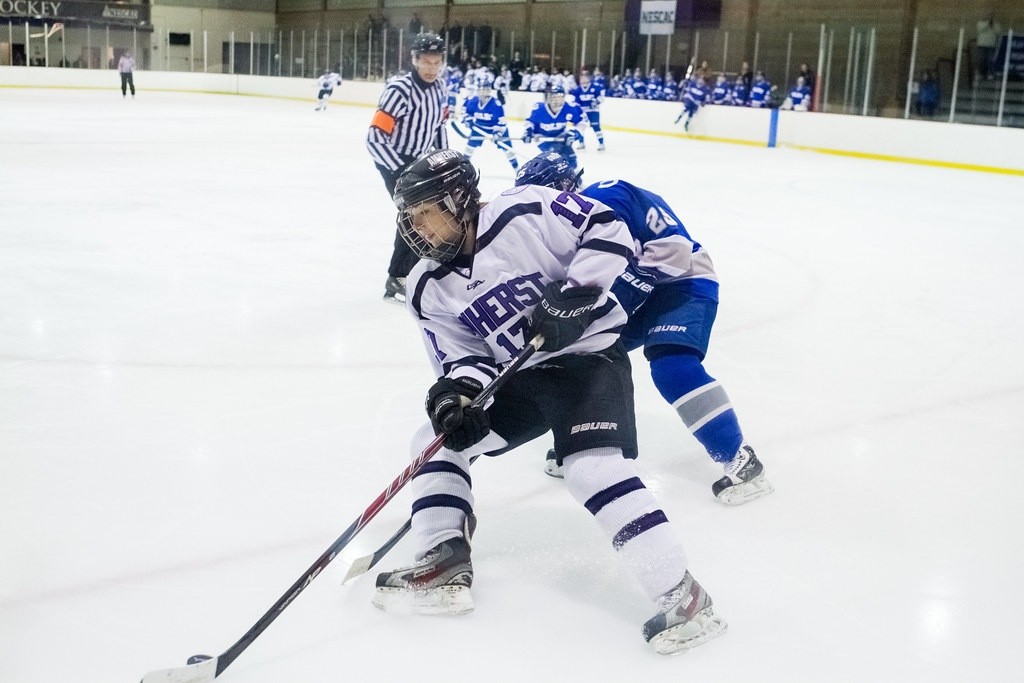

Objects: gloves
[527,279,602,354]
[425,378,490,452]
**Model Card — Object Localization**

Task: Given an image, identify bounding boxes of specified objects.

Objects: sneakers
[712,438,775,506]
[641,570,727,656]
[383,276,407,306]
[372,537,475,616]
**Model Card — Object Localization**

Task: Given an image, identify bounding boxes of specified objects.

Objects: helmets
[546,85,566,95]
[411,32,446,53]
[513,151,582,191]
[391,149,481,265]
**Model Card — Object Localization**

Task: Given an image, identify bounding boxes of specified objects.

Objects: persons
[335,14,492,77]
[371,149,726,653]
[674,74,707,131]
[915,72,940,115]
[462,80,520,173]
[315,69,341,110]
[569,73,604,151]
[443,48,816,120]
[975,13,1003,81]
[523,85,585,189]
[517,152,774,506]
[118,53,135,96]
[33,52,87,68]
[367,32,450,307]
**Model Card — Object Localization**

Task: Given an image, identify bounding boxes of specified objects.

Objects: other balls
[186,654,214,665]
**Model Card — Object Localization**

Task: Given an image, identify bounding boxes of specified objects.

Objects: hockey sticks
[340,452,482,587]
[450,120,565,143]
[472,125,531,161]
[138,333,546,683]
